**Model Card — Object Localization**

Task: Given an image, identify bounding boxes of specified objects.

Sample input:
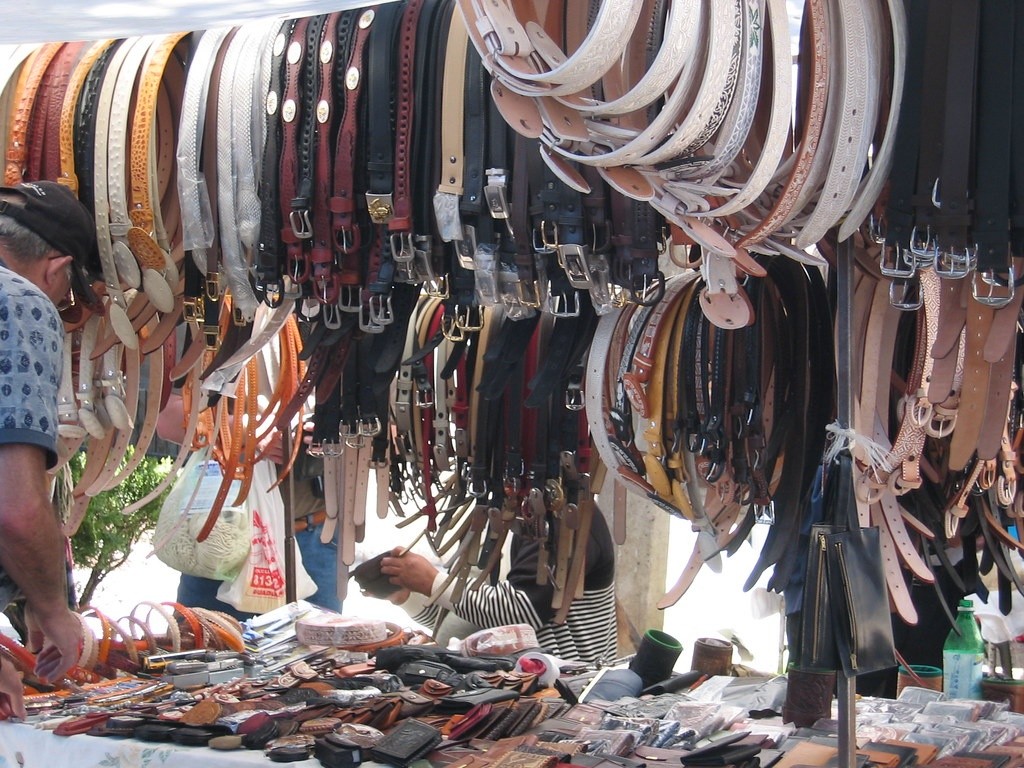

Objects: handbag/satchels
[799,453,897,679]
[152,441,322,616]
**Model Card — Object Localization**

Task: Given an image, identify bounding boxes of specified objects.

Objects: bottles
[943,599,984,701]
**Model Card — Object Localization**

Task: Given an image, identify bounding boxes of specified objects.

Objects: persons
[839,515,981,700]
[160,305,342,642]
[359,451,615,685]
[0,180,97,725]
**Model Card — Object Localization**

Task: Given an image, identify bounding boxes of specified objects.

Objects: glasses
[48,255,75,312]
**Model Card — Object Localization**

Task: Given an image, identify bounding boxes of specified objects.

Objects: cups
[896,666,942,701]
[629,629,683,687]
[692,637,732,677]
[783,665,835,725]
[983,678,1024,714]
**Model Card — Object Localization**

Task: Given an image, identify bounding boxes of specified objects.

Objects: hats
[0,180,96,305]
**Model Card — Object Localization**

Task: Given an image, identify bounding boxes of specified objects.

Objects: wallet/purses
[92,622,1023,768]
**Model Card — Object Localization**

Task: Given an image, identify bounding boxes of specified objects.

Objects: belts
[0,0,1024,691]
